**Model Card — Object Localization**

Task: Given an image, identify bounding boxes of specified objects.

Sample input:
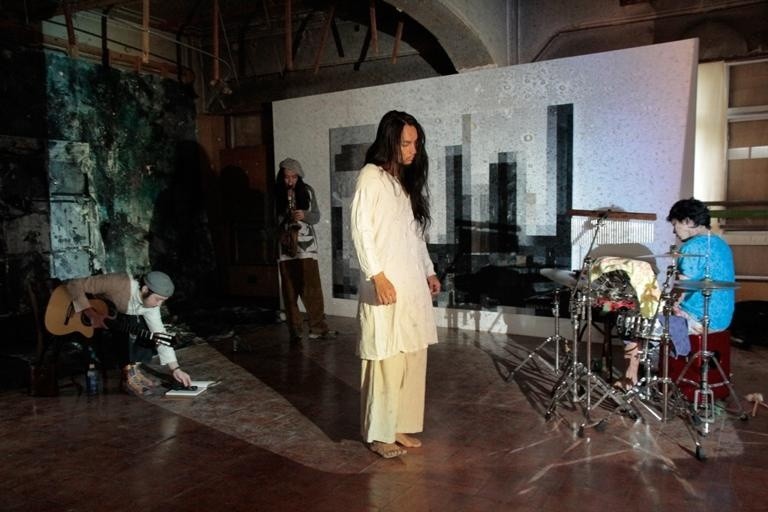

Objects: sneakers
[290,327,303,337]
[308,329,339,339]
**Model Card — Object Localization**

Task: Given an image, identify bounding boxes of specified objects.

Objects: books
[164,381,220,397]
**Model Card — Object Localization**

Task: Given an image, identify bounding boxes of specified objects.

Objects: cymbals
[673,278,743,290]
[540,265,590,294]
[635,252,707,260]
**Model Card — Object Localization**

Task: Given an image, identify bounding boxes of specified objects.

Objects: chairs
[27,279,130,372]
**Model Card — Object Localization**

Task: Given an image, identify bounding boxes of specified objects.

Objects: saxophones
[282,183,302,255]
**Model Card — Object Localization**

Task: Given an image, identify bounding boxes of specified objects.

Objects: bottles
[87,364,98,394]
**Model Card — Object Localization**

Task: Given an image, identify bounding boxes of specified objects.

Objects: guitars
[44,284,177,350]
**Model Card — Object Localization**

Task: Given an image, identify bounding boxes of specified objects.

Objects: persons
[612,197,736,391]
[66,271,192,395]
[275,158,328,340]
[348,110,441,459]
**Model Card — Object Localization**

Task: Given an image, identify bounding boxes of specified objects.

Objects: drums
[615,309,663,340]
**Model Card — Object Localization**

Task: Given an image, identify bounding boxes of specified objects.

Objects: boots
[122,365,143,397]
[134,362,162,388]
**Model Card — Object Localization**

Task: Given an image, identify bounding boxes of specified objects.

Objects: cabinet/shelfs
[719,61,767,306]
[223,106,277,297]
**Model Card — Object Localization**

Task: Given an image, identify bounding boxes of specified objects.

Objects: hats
[279,158,305,178]
[143,272,175,296]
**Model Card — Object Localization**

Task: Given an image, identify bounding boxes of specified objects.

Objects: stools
[658,332,732,402]
